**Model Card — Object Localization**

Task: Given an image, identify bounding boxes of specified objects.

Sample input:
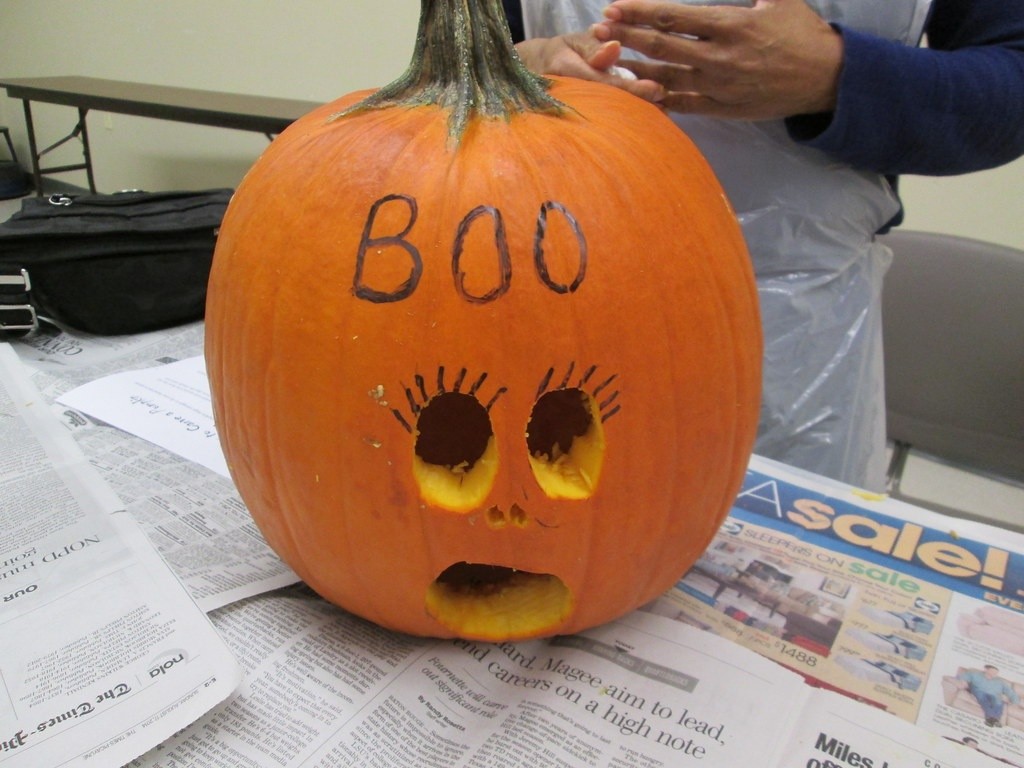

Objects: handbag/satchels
[0,187,234,337]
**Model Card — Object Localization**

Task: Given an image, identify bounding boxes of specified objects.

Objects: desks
[0,75,1024,768]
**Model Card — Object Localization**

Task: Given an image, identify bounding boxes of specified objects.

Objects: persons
[957,665,1024,729]
[500,0,1024,496]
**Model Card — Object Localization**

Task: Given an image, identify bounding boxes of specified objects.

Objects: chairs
[875,229,1024,536]
